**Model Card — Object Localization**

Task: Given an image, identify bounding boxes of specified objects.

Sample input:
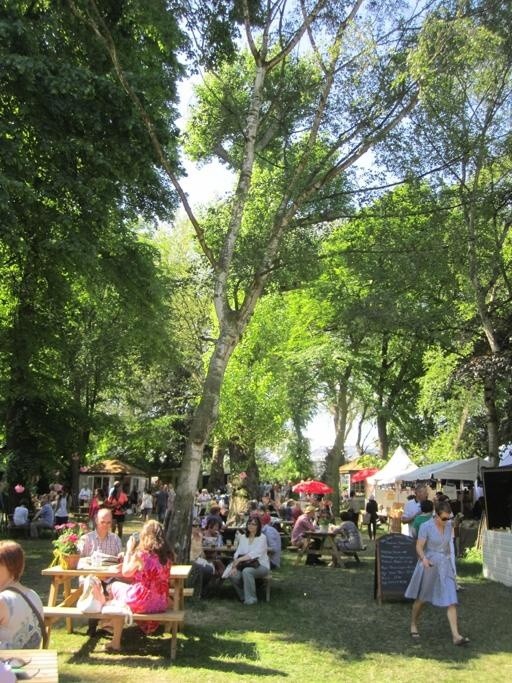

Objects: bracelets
[418,557,427,563]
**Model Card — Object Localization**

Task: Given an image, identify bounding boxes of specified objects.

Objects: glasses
[438,513,454,521]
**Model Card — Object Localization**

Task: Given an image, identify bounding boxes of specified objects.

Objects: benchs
[63,588,194,598]
[42,607,185,661]
[195,569,271,604]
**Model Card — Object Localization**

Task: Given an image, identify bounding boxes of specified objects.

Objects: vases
[59,553,80,570]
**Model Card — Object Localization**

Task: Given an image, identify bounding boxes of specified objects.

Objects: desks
[1,648,59,683]
[202,545,273,555]
[41,557,193,637]
[304,527,338,565]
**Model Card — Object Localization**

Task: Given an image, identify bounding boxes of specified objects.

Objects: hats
[261,514,270,523]
[368,495,374,500]
[304,506,319,514]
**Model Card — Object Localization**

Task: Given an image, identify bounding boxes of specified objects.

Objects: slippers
[457,636,470,647]
[409,631,420,638]
[105,643,120,654]
[101,623,113,629]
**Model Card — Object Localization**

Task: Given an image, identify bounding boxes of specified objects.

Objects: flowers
[51,524,85,569]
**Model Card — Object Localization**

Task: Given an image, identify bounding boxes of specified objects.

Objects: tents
[395,458,493,556]
[366,441,421,485]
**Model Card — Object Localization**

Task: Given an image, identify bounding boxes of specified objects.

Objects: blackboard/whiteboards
[374,533,418,606]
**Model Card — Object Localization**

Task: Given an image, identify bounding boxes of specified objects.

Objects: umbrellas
[292,480,334,495]
[352,467,379,484]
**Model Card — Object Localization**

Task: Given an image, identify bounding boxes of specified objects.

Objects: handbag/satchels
[120,501,131,512]
[363,512,371,525]
[101,599,133,626]
[236,554,260,570]
[77,575,105,615]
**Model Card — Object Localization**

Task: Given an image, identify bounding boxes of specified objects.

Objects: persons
[13,498,30,526]
[328,512,361,568]
[399,485,429,535]
[260,515,281,570]
[257,481,283,515]
[439,494,450,503]
[366,495,377,541]
[347,491,357,511]
[192,487,232,511]
[404,501,471,646]
[106,482,130,537]
[106,519,173,652]
[290,506,326,567]
[412,500,435,539]
[285,499,295,519]
[454,511,464,557]
[243,499,259,513]
[89,490,103,519]
[0,539,47,649]
[292,503,302,520]
[191,519,215,597]
[202,516,225,577]
[229,515,270,605]
[78,484,92,504]
[75,509,125,636]
[435,492,443,501]
[55,487,71,524]
[30,498,56,539]
[137,481,177,522]
[204,507,226,532]
